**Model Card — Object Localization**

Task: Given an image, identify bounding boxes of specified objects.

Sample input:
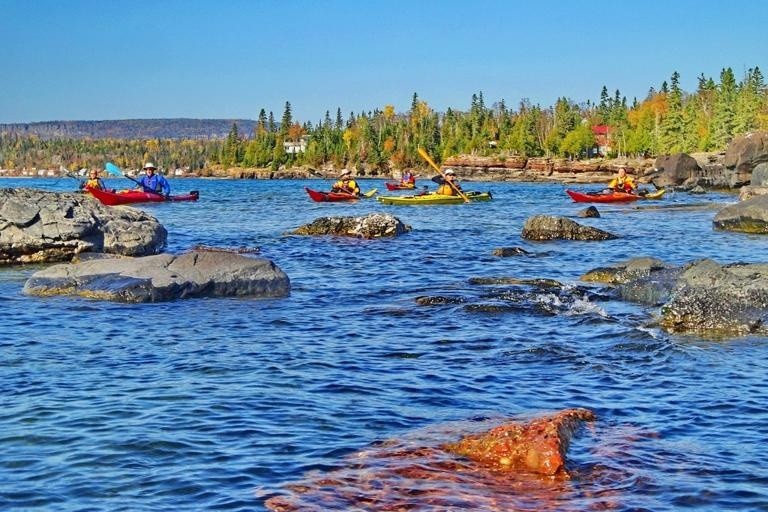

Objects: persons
[608,166,638,196]
[136,162,171,198]
[405,171,415,185]
[430,169,464,196]
[79,168,106,192]
[332,167,360,197]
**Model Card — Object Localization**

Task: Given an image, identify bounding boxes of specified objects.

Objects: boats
[87,185,199,206]
[376,191,493,206]
[384,182,418,191]
[304,186,377,202]
[565,187,667,203]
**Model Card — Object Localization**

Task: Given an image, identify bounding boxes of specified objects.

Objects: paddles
[417,146,470,202]
[104,162,173,201]
[305,168,361,201]
[59,166,86,184]
[587,167,665,195]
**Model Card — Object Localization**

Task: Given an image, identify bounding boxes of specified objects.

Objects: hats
[340,169,352,176]
[445,169,457,175]
[143,163,157,170]
